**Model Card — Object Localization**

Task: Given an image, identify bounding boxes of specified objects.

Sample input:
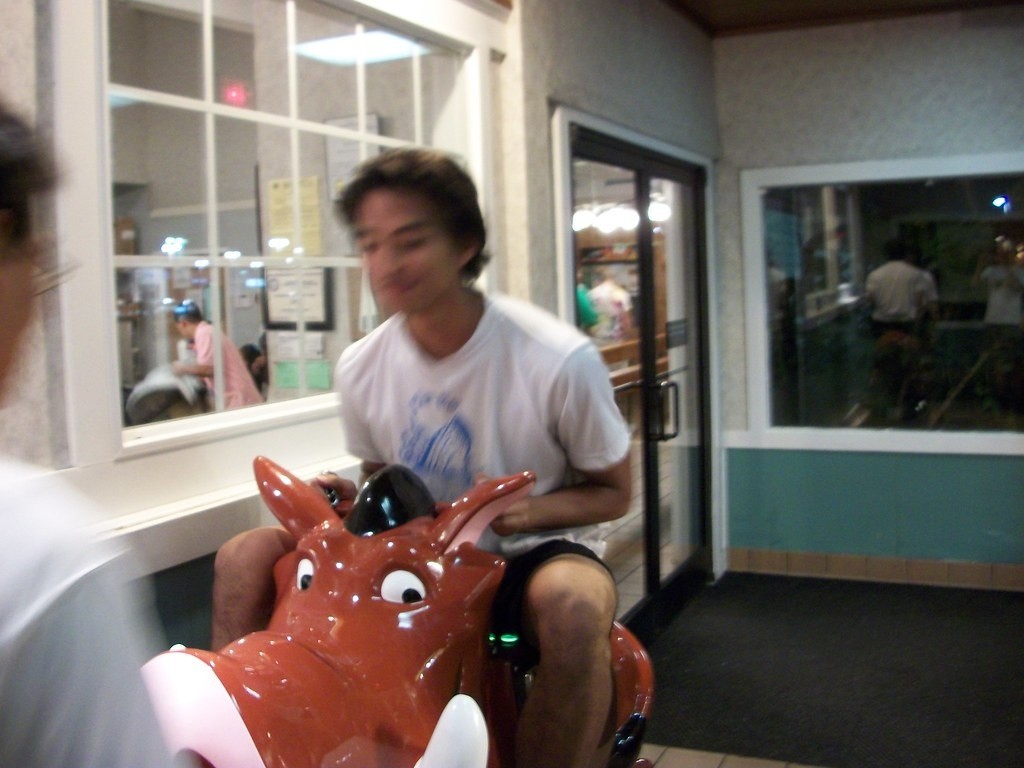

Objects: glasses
[17,243,64,296]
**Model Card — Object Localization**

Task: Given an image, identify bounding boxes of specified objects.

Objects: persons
[860,237,1024,415]
[573,271,637,368]
[172,298,270,410]
[208,148,634,768]
[0,101,186,768]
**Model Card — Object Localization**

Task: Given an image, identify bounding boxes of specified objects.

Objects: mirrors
[741,152,1024,456]
